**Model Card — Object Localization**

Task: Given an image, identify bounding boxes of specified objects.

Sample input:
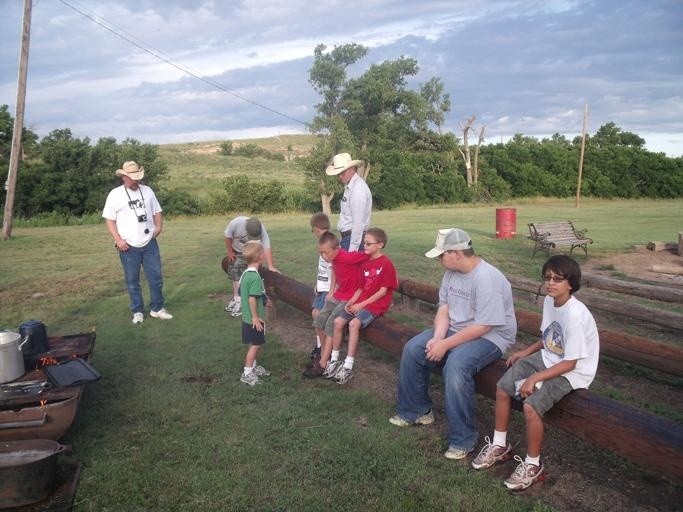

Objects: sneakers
[224,299,234,312]
[388,410,436,427]
[239,372,263,385]
[333,368,354,386]
[471,443,513,471]
[131,312,144,325]
[502,463,545,492]
[303,364,324,378]
[251,366,270,376]
[231,302,241,317]
[444,445,474,459]
[322,360,343,379]
[308,346,321,363]
[148,308,173,320]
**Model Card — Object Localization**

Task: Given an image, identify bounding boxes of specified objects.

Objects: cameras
[138,214,148,222]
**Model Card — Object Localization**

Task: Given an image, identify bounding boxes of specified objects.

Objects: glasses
[541,272,567,283]
[363,240,377,246]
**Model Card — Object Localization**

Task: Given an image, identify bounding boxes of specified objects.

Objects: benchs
[527,220,592,259]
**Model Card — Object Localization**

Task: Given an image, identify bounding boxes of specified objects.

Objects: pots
[18,319,49,355]
[0,331,29,384]
[0,439,66,508]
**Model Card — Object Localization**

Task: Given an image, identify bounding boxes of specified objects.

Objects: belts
[339,230,351,237]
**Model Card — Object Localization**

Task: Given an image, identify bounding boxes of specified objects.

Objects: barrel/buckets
[495,206,517,238]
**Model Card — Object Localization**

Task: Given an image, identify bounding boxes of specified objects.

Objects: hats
[114,160,144,181]
[424,227,474,259]
[324,152,364,177]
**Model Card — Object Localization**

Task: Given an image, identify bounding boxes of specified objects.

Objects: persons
[100,161,174,322]
[470,252,599,491]
[299,235,371,378]
[308,216,331,358]
[386,227,519,458]
[238,239,272,386]
[318,228,400,386]
[226,212,282,317]
[326,153,373,257]
